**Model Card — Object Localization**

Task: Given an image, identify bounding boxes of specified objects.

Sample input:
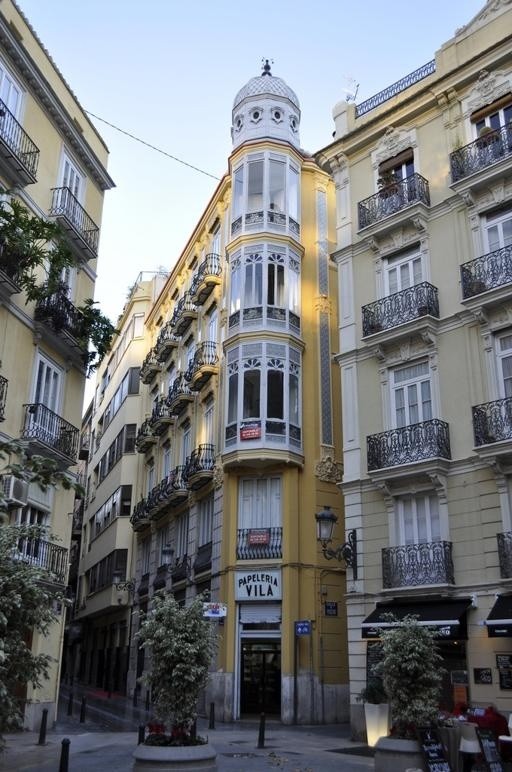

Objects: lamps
[314,504,357,580]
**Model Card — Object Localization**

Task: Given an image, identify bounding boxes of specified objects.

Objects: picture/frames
[474,668,492,684]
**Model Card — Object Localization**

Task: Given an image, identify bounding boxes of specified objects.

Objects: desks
[450,682,470,708]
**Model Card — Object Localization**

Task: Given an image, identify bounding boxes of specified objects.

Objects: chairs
[497,735,512,772]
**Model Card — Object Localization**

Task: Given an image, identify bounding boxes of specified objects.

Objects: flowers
[147,723,167,734]
[170,727,187,735]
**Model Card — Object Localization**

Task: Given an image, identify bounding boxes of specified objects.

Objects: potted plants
[367,611,451,772]
[129,584,217,772]
[355,677,390,748]
